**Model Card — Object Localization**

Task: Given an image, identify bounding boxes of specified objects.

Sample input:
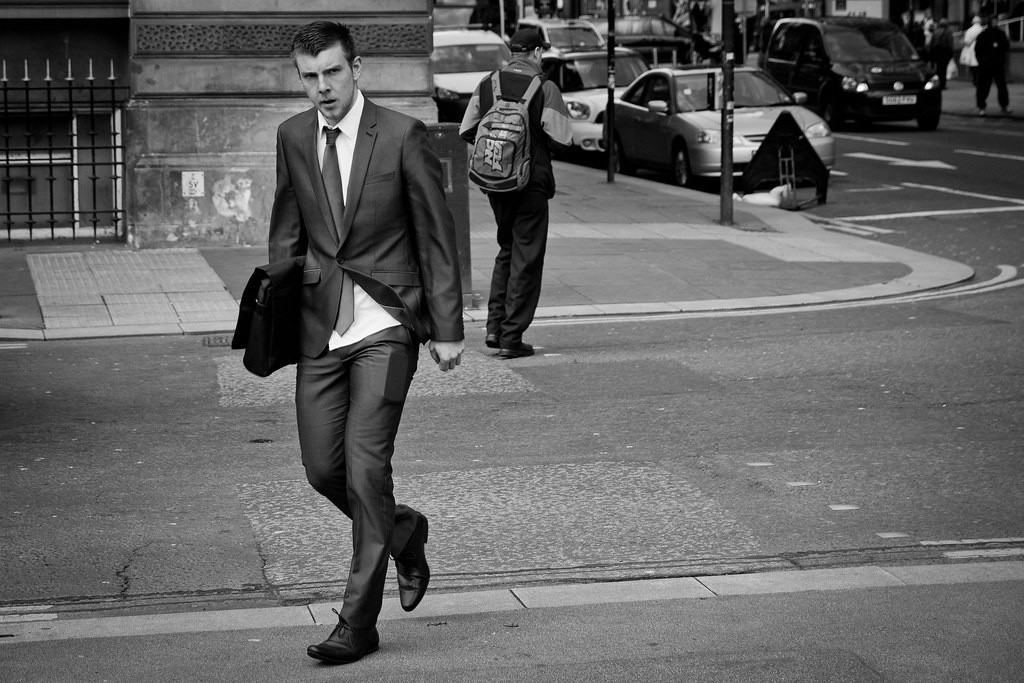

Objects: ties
[320,126,355,336]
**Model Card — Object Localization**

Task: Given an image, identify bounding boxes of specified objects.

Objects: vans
[763,14,945,133]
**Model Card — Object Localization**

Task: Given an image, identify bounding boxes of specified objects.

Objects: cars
[430,10,724,168]
[600,60,837,192]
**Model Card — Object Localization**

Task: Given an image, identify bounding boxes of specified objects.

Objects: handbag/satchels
[965,22,989,44]
[230,254,307,378]
[947,59,959,81]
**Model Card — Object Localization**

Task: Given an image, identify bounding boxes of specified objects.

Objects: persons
[901,9,987,89]
[975,12,1012,114]
[268,20,467,663]
[456,31,574,359]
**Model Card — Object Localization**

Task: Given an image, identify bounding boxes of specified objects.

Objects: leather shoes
[499,342,534,356]
[388,511,431,611]
[308,609,379,664]
[485,333,499,348]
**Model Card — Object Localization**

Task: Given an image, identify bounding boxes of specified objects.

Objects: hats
[509,29,551,54]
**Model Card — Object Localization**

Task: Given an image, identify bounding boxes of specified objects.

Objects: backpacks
[467,70,546,192]
